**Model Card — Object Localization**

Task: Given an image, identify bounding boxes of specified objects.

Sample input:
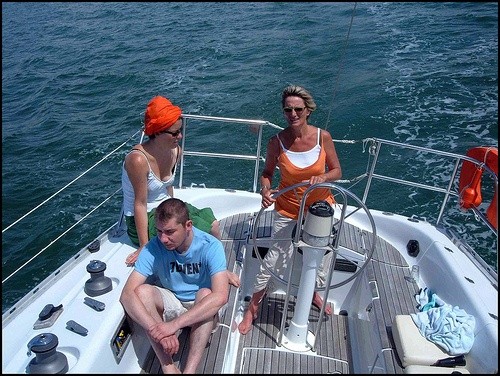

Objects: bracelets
[260,185,268,195]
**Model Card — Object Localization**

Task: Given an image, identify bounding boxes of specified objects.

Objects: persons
[119,198,230,374]
[238,86,342,336]
[121,95,242,287]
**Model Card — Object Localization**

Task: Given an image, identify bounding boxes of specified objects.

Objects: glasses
[163,127,183,136]
[283,106,307,112]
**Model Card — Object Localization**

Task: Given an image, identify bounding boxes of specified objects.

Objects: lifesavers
[460,147,498,229]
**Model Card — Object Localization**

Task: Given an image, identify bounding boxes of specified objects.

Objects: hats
[145,96,182,135]
